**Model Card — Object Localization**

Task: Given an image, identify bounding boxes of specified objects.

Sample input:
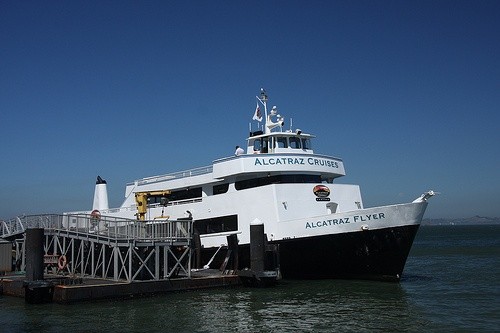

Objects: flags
[252,99,263,122]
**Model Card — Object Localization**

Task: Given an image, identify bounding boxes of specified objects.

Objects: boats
[61,89,435,281]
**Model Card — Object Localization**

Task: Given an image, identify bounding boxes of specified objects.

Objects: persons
[235,146,245,155]
